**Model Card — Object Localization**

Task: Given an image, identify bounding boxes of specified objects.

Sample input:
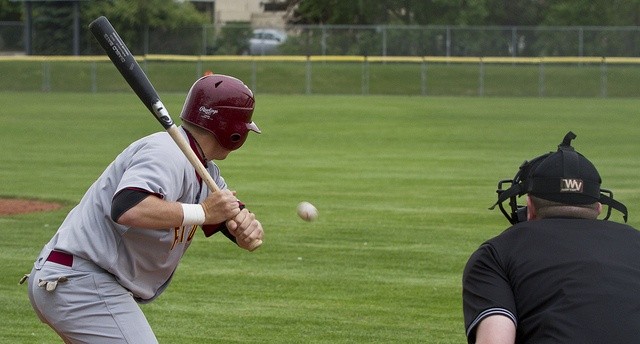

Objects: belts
[44,249,74,268]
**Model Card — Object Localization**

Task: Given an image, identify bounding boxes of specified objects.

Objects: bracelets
[179,201,205,226]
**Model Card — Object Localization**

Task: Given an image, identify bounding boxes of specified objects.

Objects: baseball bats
[88,15,262,252]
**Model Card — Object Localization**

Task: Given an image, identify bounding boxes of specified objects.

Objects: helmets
[180,74,262,150]
[489,131,627,220]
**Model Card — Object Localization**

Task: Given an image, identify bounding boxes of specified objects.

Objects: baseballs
[297,202,317,222]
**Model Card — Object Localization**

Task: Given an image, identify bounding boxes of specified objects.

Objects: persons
[461,130,640,343]
[27,74,260,344]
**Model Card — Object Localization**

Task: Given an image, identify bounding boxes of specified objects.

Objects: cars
[242,29,287,55]
